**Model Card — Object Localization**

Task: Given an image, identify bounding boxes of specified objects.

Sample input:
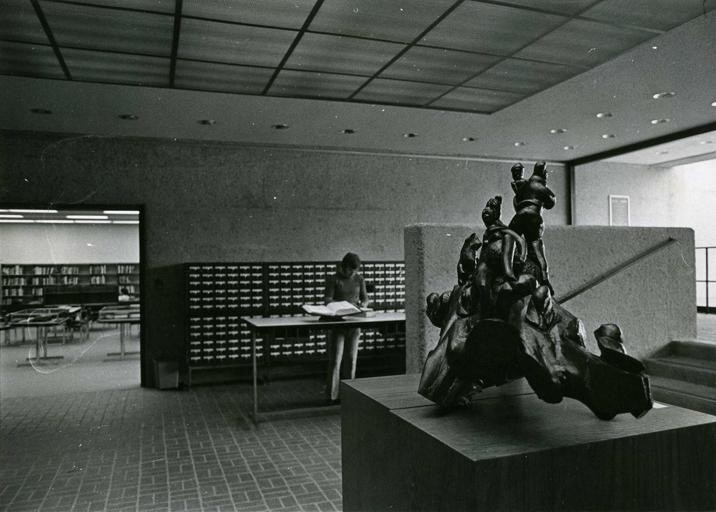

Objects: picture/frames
[609,196,630,227]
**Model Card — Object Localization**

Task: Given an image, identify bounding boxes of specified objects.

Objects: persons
[324,250,369,406]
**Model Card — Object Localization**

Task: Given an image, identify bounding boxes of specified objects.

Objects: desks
[243,313,406,421]
[1,301,141,364]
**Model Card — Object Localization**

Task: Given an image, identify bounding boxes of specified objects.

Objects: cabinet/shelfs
[0,263,139,306]
[167,262,406,386]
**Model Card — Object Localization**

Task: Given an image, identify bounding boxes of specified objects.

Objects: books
[0,263,139,322]
[302,299,362,316]
